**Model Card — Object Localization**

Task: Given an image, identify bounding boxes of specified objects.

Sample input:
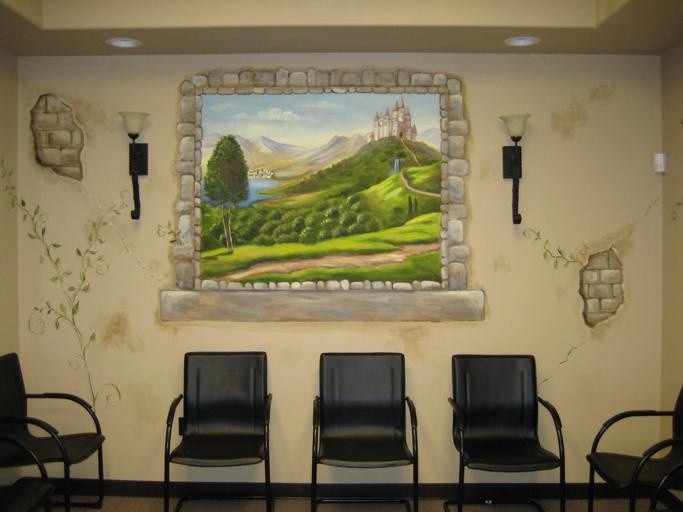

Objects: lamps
[118,112,151,220]
[499,113,532,224]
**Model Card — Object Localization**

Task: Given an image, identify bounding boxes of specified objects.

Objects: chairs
[443,353,566,512]
[164,352,275,512]
[311,352,419,512]
[586,384,683,512]
[648,461,683,512]
[0,352,105,512]
[0,432,56,512]
